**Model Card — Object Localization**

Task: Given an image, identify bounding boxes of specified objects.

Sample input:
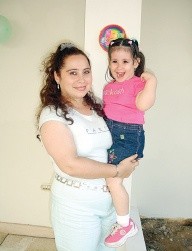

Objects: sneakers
[103,217,138,247]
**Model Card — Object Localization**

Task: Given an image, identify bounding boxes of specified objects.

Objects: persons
[35,43,147,251]
[102,38,158,248]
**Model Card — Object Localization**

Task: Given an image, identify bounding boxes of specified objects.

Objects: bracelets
[113,164,119,177]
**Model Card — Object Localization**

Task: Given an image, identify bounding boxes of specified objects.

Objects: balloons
[0,15,13,45]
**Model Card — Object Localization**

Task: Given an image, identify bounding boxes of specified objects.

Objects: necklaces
[61,93,83,102]
